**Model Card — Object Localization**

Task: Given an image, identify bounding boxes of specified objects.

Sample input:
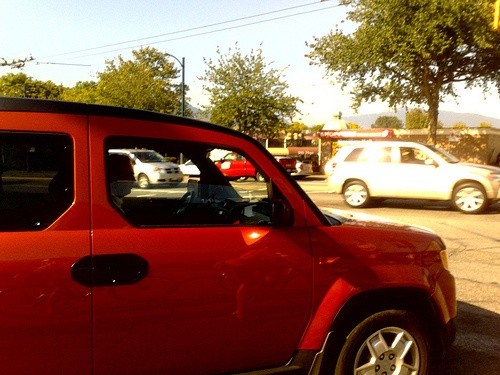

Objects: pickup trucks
[212,152,300,184]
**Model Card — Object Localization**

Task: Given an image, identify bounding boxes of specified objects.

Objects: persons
[107,152,178,225]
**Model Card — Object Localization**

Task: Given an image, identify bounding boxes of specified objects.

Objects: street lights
[164,53,186,116]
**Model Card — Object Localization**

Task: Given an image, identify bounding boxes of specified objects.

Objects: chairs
[408,149,416,160]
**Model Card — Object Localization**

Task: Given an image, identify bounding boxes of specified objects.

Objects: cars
[324,141,499,215]
[107,147,183,190]
[0,97,458,375]
[274,155,313,178]
[176,158,214,175]
